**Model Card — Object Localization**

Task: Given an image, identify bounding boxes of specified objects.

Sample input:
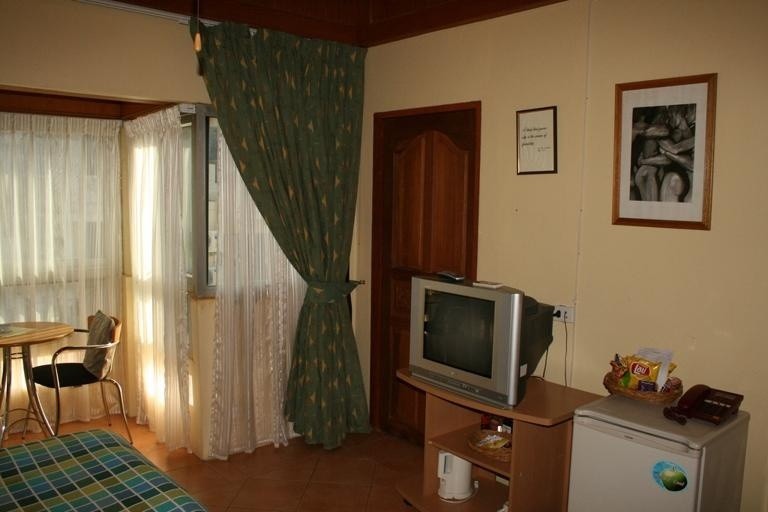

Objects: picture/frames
[610,71,718,231]
[516,105,558,175]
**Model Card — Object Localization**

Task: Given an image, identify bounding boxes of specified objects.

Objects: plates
[439,479,478,502]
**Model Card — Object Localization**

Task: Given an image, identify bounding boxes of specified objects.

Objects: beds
[0,428,206,512]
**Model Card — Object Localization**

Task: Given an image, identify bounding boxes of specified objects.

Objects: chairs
[21,314,134,446]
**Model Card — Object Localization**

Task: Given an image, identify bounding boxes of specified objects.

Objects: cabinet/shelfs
[394,367,604,512]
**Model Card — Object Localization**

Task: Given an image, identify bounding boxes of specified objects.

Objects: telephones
[664,383,745,425]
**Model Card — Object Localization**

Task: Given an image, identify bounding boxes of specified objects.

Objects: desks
[0,321,75,446]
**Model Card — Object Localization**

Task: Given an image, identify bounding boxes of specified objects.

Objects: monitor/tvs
[408,274,556,412]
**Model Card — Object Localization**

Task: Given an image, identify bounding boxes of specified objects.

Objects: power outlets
[552,304,576,325]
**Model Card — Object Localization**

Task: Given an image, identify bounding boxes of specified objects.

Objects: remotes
[472,280,502,290]
[437,268,465,281]
